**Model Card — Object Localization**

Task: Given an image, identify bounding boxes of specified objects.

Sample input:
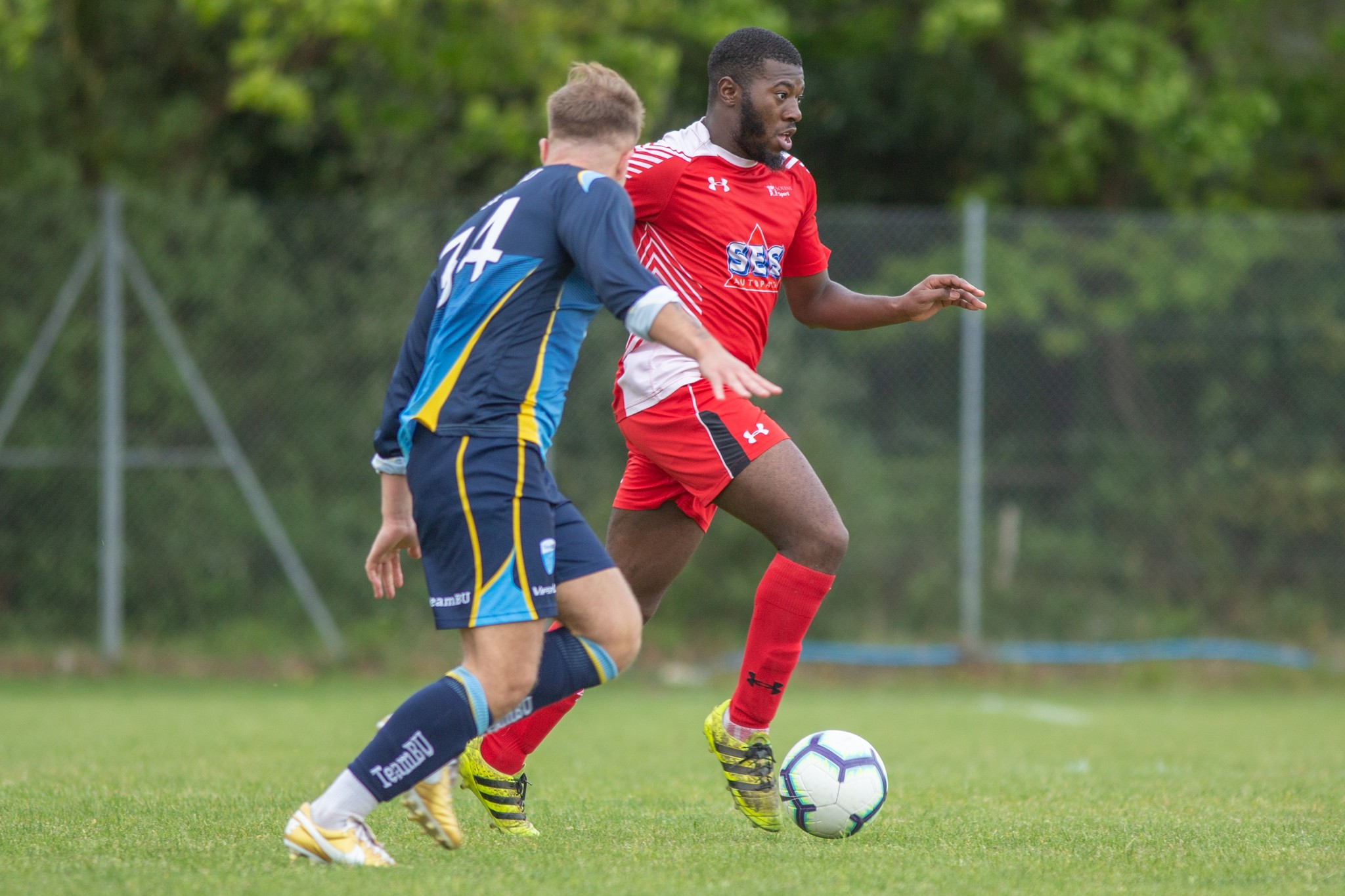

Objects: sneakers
[458,736,539,838]
[703,699,784,833]
[283,801,395,868]
[376,712,462,850]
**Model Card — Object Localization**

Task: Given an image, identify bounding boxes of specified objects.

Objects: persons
[455,27,987,841]
[283,61,783,873]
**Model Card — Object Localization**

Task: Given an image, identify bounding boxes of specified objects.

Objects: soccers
[779,731,889,839]
